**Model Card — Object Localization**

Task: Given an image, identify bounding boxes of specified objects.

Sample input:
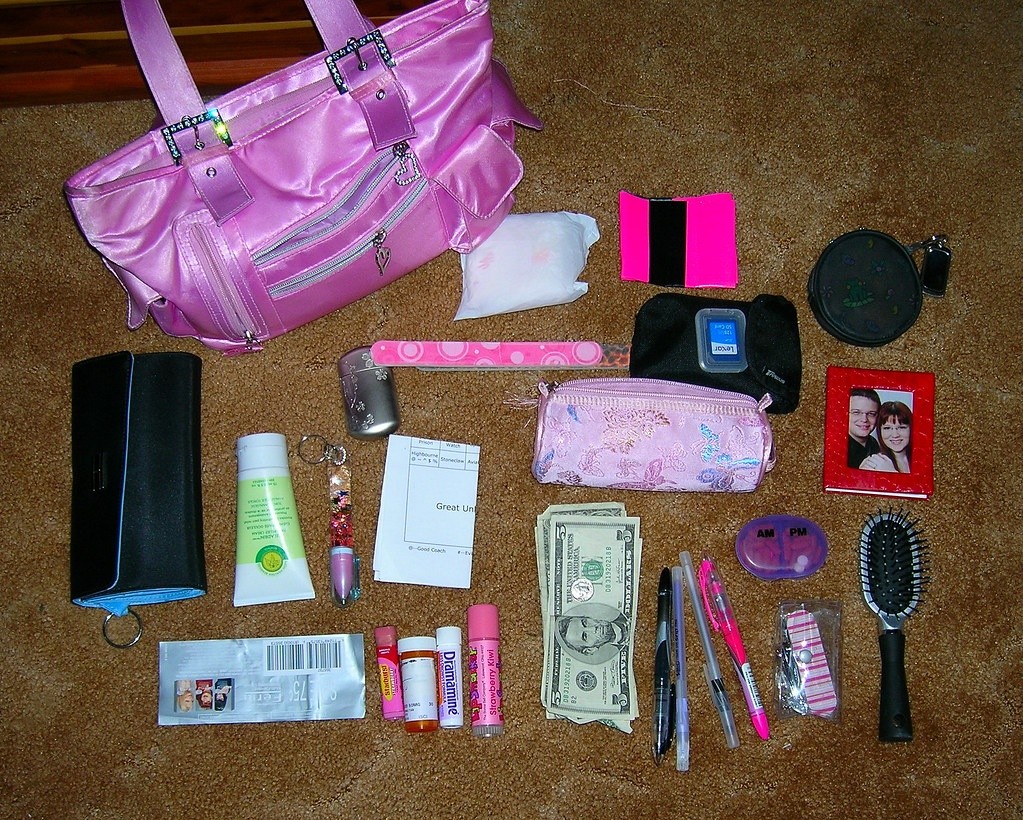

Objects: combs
[855,505,934,745]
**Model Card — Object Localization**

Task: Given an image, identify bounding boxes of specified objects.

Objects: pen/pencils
[649,550,769,772]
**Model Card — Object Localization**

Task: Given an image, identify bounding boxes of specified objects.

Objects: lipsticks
[327,460,362,609]
[466,602,505,740]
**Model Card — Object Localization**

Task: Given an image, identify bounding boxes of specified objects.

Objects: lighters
[337,344,401,443]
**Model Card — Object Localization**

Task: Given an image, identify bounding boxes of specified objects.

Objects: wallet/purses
[68,352,208,649]
[616,187,740,290]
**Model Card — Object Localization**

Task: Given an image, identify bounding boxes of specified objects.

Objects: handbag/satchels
[60,1,541,353]
[808,225,924,346]
[627,293,802,416]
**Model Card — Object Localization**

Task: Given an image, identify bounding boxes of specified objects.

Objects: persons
[858,400,912,474]
[176,677,233,713]
[846,387,881,470]
[556,613,631,656]
